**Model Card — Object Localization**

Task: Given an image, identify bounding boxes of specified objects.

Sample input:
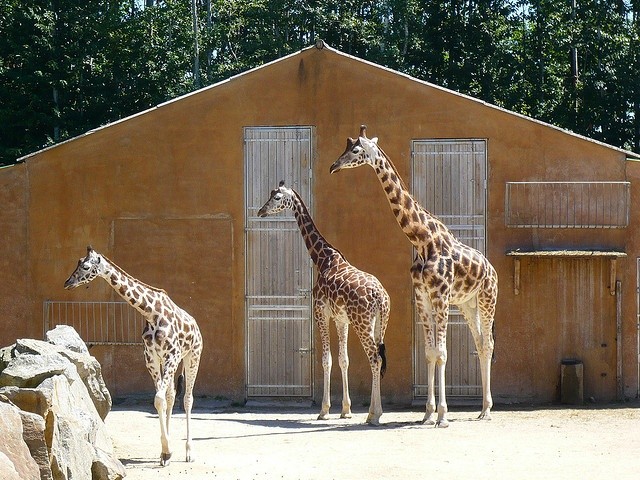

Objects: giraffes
[62,244,206,468]
[328,124,502,429]
[257,178,392,427]
